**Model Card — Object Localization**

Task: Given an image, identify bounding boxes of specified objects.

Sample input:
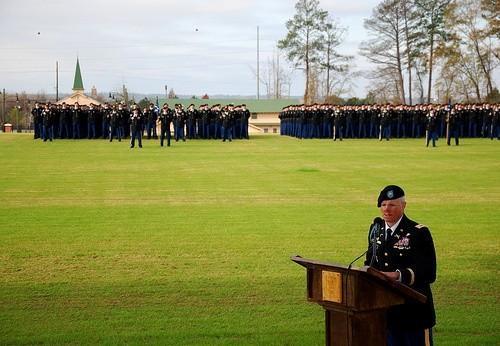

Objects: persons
[278,100,500,140]
[128,108,143,148]
[445,107,460,146]
[32,101,250,143]
[157,107,173,147]
[364,185,437,346]
[423,108,437,147]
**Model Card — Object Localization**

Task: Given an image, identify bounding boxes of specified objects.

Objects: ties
[387,228,392,242]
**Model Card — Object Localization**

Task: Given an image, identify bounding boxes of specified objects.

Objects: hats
[378,185,405,207]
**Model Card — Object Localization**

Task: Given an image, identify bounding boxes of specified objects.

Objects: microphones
[348,234,384,271]
[374,217,383,238]
